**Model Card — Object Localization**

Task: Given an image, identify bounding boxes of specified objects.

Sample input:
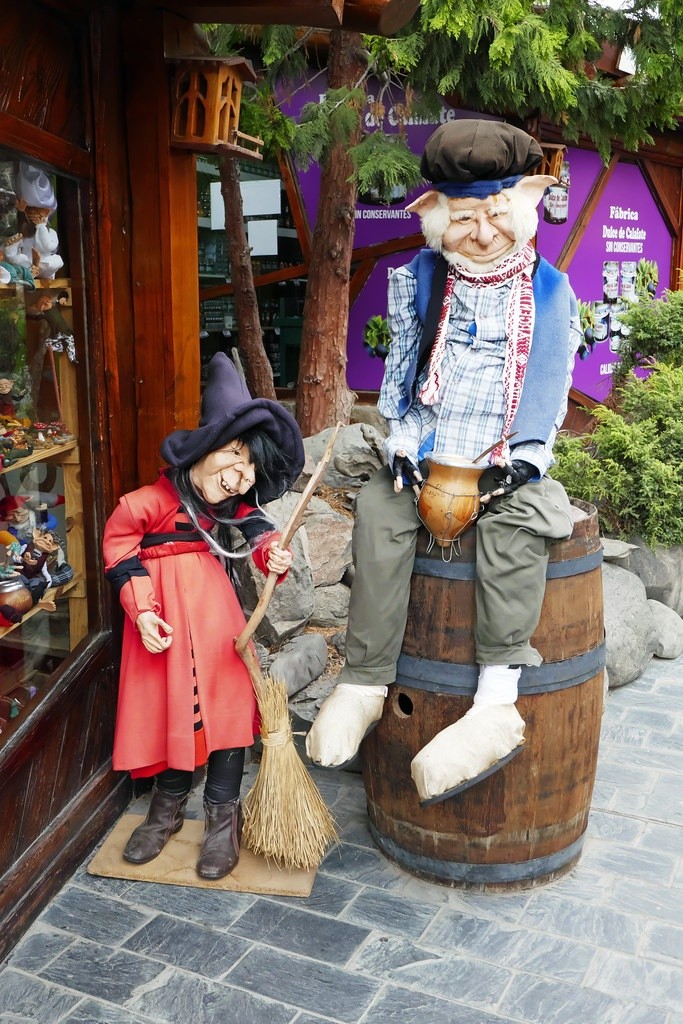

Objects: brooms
[233,420,346,872]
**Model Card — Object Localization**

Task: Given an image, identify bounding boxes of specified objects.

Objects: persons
[0,494,60,612]
[0,379,31,419]
[25,290,81,366]
[304,121,584,805]
[103,352,305,881]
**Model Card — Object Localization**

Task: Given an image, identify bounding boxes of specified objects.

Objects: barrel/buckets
[356,498,607,892]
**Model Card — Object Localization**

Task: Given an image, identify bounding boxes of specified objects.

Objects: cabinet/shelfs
[0,278,89,701]
